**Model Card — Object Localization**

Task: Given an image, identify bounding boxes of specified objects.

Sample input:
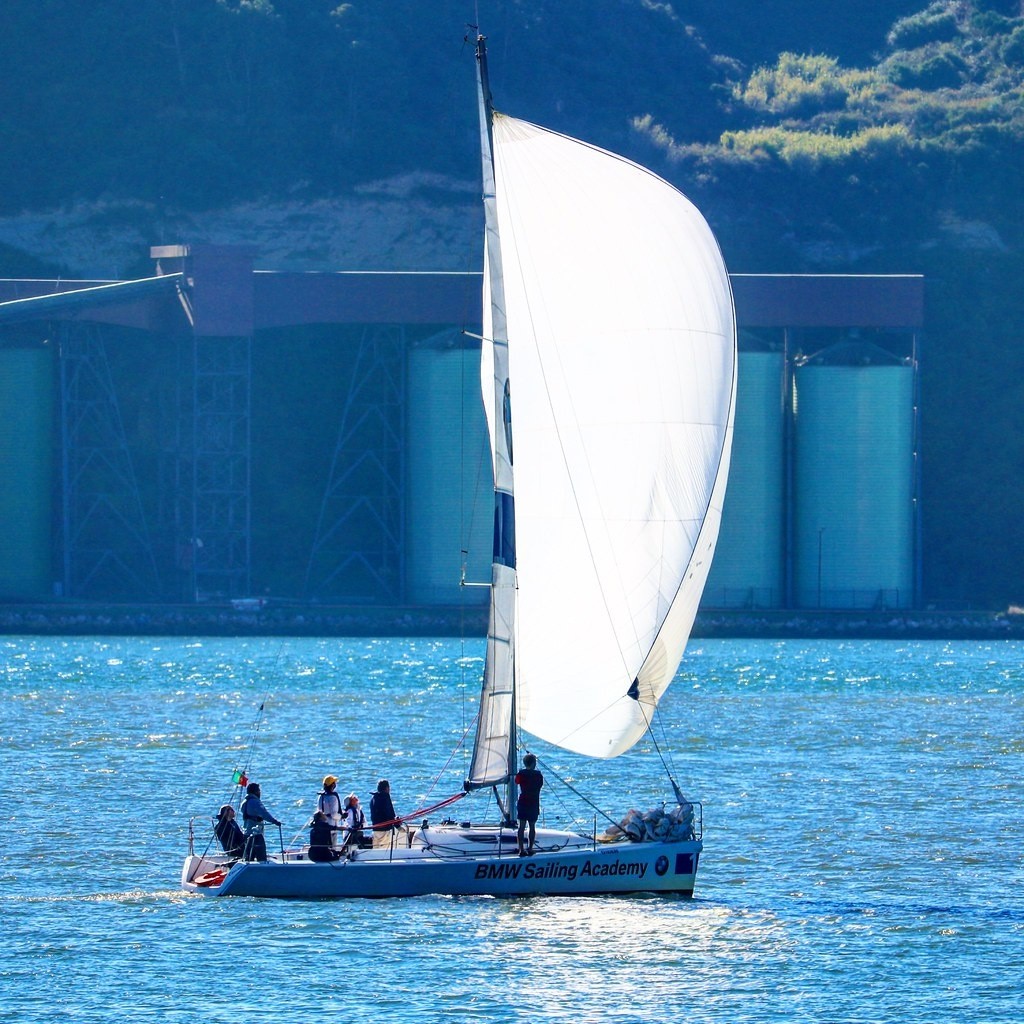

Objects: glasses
[228,809,235,812]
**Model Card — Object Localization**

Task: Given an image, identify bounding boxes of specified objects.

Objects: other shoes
[527,850,536,856]
[519,852,527,857]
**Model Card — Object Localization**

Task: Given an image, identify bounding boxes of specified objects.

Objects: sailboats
[181,33,739,902]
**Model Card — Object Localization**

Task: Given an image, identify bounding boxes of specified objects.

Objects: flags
[232,772,248,786]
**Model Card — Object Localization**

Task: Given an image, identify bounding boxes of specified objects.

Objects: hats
[323,776,338,785]
[343,792,354,808]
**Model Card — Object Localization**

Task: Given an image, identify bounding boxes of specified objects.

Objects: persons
[317,776,348,850]
[341,792,368,842]
[308,811,346,862]
[241,783,281,861]
[214,805,246,868]
[370,780,405,849]
[515,755,543,857]
